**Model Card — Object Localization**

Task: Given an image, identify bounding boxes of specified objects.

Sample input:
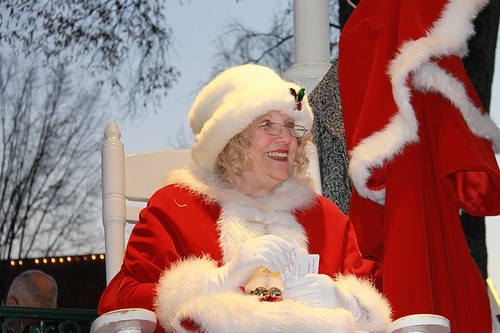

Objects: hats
[188,63,314,174]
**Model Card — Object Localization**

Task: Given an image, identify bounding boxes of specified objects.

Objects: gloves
[200,234,294,294]
[282,273,362,322]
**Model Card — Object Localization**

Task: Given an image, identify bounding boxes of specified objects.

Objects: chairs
[101,122,323,289]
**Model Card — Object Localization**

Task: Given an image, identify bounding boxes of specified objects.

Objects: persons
[98,62,392,333]
[5,271,62,332]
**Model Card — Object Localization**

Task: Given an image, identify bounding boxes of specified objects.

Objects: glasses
[250,122,308,138]
